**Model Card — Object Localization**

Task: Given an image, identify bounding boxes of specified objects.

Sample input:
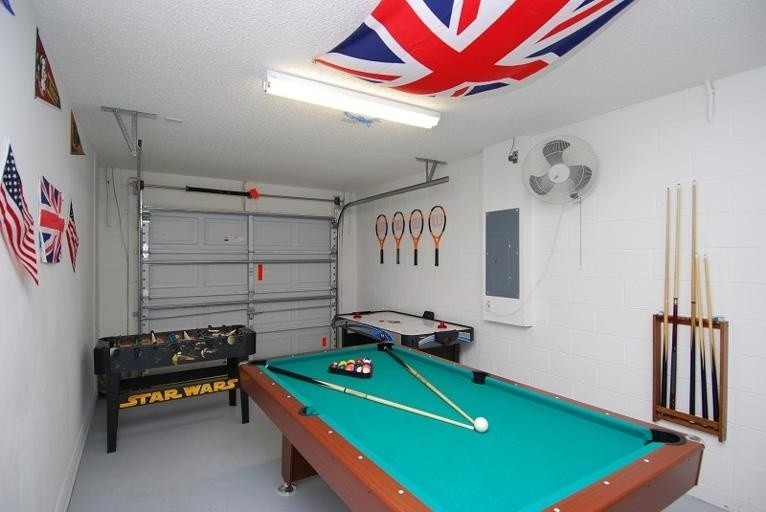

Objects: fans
[522,134,601,205]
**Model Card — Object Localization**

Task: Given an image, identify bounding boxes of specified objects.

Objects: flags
[63,199,82,273]
[0,142,42,288]
[37,176,63,266]
[309,0,637,99]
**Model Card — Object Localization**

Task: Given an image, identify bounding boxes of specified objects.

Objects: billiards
[332,358,371,374]
[474,417,488,432]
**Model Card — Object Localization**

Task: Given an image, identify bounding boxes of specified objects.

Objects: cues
[384,346,475,424]
[267,365,474,430]
[663,180,719,422]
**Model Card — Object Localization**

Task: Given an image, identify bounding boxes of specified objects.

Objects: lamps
[262,70,442,129]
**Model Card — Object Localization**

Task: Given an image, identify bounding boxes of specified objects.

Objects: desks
[237,341,705,512]
[95,325,256,453]
[332,310,474,367]
